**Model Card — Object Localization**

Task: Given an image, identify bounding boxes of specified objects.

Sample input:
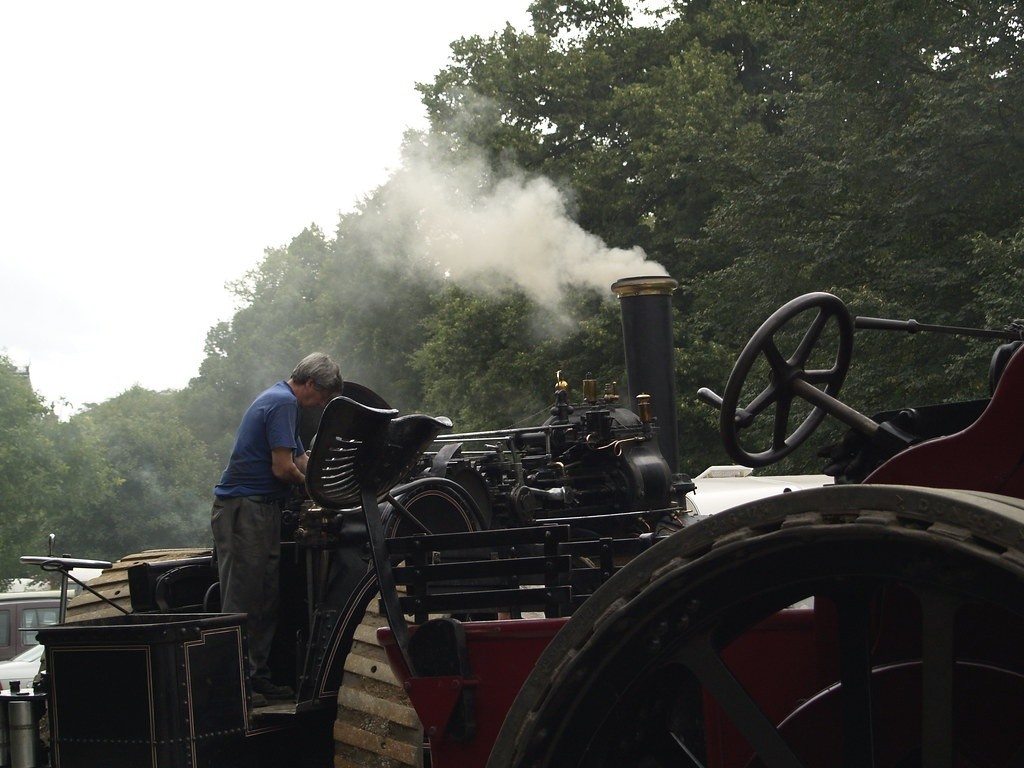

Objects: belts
[247,494,275,504]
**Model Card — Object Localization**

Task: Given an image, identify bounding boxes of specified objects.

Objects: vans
[0,588,76,661]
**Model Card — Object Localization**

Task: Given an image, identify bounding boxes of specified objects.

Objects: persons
[211,352,342,707]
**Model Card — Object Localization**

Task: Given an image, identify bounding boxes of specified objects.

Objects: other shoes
[248,683,268,706]
[253,679,296,699]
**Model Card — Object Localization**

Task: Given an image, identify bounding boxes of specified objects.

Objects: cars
[0,643,44,690]
